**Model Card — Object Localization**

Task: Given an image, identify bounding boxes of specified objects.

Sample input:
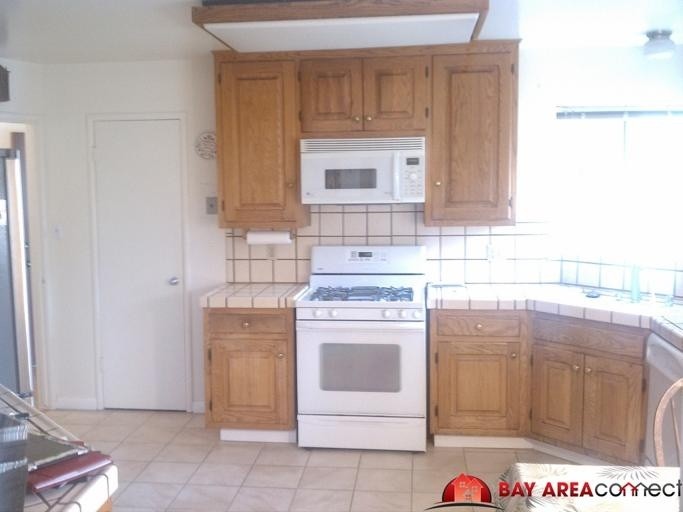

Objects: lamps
[644,30,677,60]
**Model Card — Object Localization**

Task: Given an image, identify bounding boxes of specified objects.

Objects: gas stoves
[309,284,415,303]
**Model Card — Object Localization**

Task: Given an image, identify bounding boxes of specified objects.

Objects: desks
[496,464,683,512]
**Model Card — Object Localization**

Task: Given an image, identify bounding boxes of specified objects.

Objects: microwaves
[298,134,426,208]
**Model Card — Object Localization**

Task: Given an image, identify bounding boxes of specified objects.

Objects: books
[26,437,114,496]
[25,430,83,473]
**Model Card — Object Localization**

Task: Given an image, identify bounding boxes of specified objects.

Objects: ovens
[294,308,426,419]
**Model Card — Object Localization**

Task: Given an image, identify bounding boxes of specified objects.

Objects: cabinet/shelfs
[529,310,653,465]
[298,45,428,138]
[213,49,311,230]
[204,308,296,444]
[429,310,529,450]
[425,39,519,226]
